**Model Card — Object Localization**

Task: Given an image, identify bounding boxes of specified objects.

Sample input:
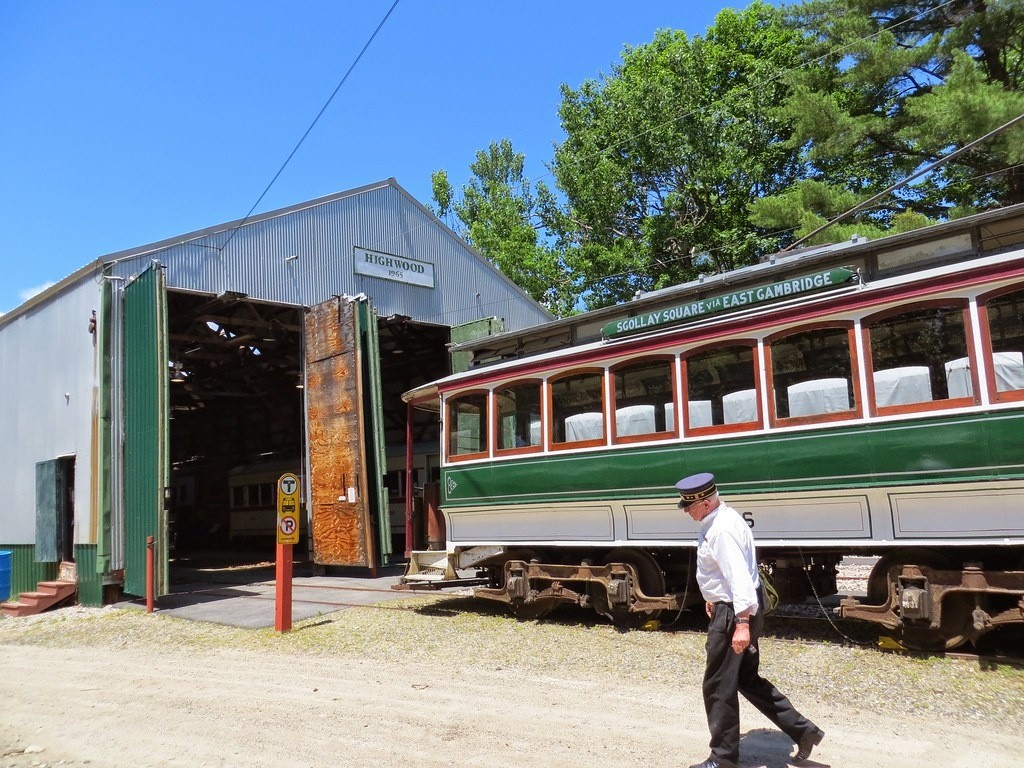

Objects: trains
[386,199,1023,660]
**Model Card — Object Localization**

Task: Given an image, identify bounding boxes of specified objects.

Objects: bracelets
[734,617,749,624]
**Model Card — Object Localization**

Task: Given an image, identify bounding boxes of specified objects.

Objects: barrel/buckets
[0,550,12,601]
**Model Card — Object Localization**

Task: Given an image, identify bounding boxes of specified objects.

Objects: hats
[675,473,717,508]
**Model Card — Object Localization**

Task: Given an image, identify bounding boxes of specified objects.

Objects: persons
[676,473,824,768]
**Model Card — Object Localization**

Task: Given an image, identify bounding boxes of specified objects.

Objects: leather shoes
[791,729,825,764]
[689,757,720,768]
